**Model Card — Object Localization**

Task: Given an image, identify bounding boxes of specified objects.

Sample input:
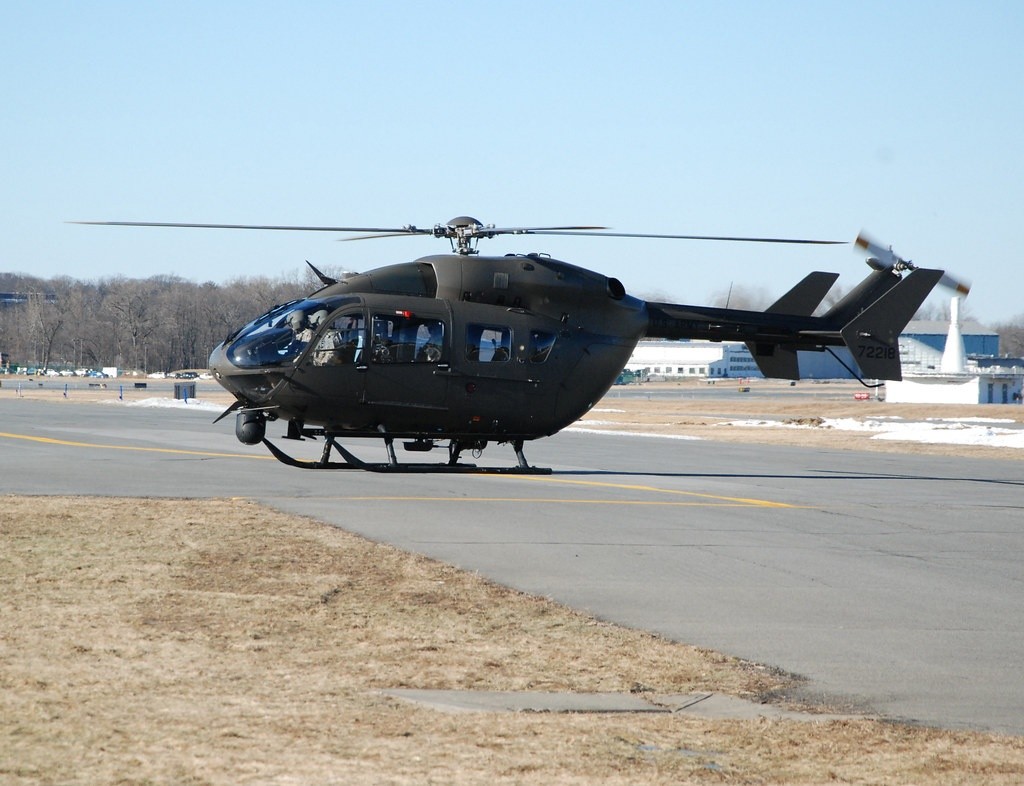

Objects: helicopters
[69,210,978,476]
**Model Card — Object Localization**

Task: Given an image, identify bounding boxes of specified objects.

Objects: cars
[0,367,108,378]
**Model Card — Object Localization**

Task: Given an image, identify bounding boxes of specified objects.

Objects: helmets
[285,310,308,330]
[310,310,329,328]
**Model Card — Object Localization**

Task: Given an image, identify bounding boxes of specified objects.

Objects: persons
[286,311,342,365]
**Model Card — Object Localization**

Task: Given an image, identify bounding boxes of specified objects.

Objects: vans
[146,372,168,379]
